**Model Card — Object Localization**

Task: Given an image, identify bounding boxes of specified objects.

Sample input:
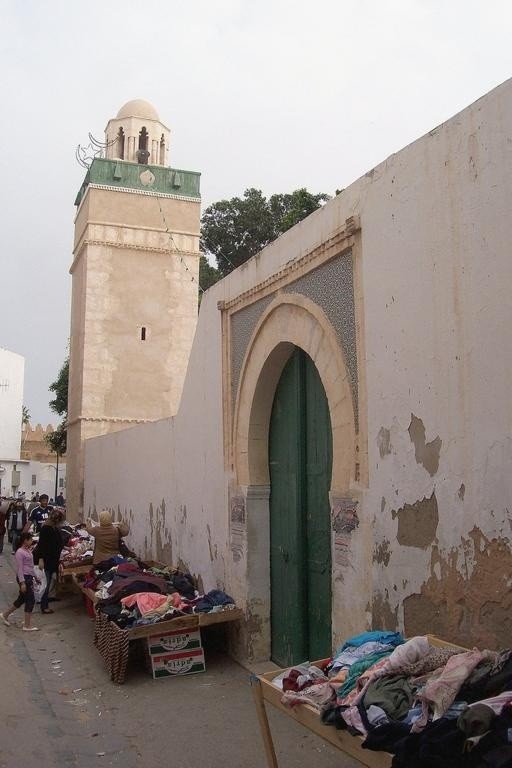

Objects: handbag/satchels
[119,538,130,555]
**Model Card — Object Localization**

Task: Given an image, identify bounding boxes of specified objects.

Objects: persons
[32,507,75,614]
[84,510,130,565]
[0,530,43,631]
[0,490,65,553]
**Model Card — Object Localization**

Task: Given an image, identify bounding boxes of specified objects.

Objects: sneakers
[23,626,39,631]
[48,597,60,602]
[41,608,54,614]
[0,613,10,626]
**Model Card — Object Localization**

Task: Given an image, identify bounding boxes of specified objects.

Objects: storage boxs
[142,627,207,679]
